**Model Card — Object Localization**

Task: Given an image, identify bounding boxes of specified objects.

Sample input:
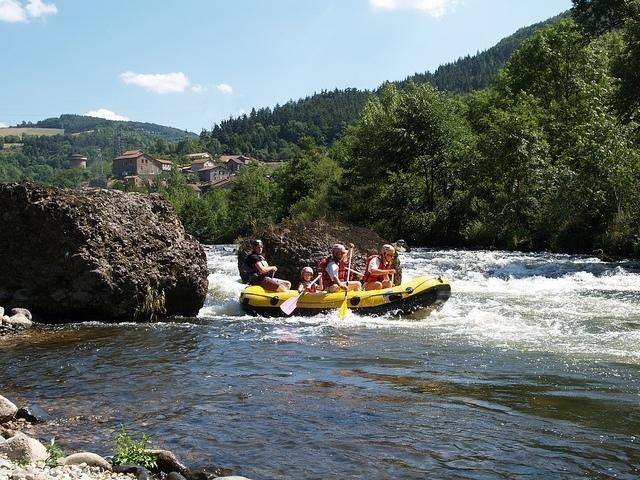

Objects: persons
[297,265,325,295]
[319,243,361,295]
[360,244,397,291]
[245,237,291,292]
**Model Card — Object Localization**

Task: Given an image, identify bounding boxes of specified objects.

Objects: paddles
[279,274,322,315]
[340,247,353,320]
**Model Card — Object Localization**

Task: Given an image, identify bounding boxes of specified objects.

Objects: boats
[239,275,451,319]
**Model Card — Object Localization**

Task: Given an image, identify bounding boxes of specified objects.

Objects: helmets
[251,239,263,250]
[301,266,314,276]
[381,244,395,259]
[333,244,346,256]
